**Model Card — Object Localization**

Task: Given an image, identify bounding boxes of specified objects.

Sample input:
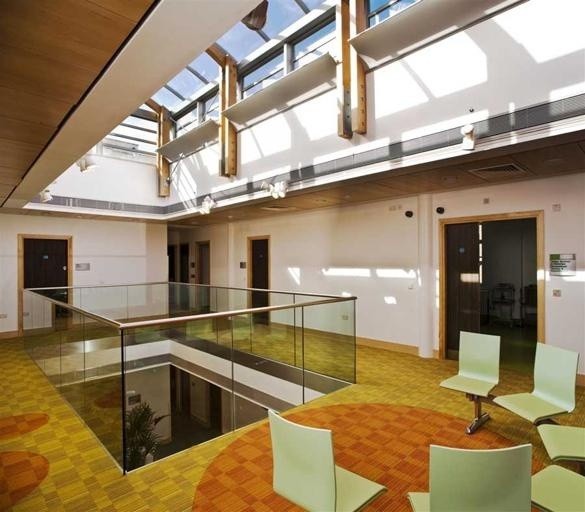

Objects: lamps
[259,179,289,201]
[197,194,219,216]
[459,124,476,151]
[72,148,99,175]
[37,185,55,204]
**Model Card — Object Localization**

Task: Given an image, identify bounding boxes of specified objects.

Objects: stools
[533,416,585,463]
[532,459,585,510]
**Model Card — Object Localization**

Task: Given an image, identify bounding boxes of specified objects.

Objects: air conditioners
[125,389,142,425]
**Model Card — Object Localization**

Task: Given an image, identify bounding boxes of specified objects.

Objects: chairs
[491,282,518,330]
[438,325,507,443]
[263,404,392,512]
[519,283,538,330]
[490,337,582,426]
[396,438,540,511]
